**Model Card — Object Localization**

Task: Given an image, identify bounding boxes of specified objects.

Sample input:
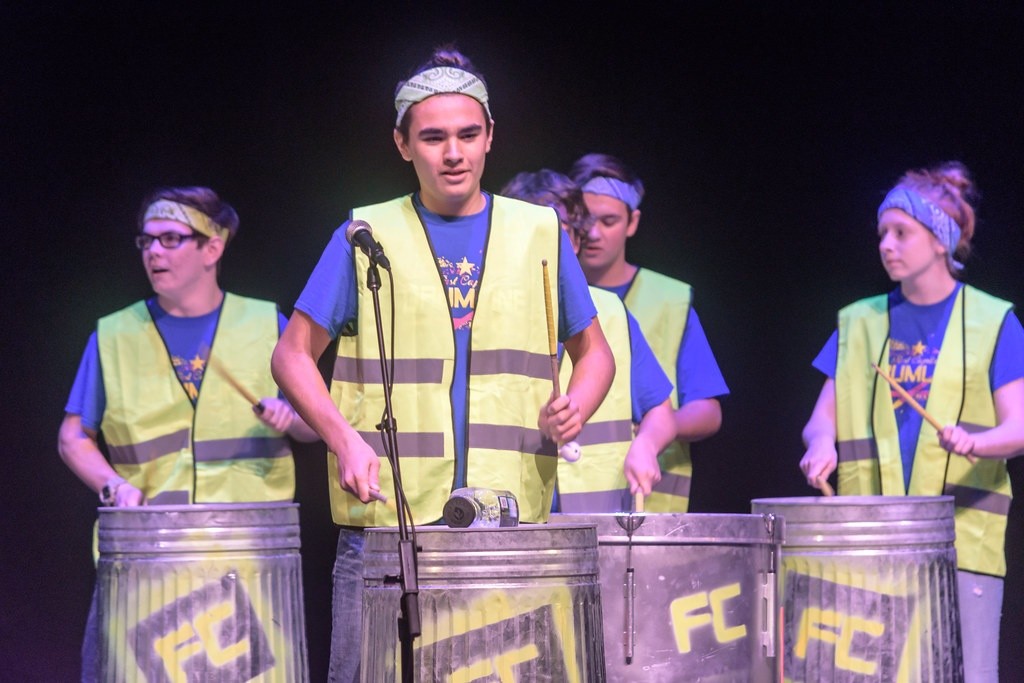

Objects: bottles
[443,487,519,527]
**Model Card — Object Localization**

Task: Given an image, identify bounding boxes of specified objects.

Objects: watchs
[98,476,127,508]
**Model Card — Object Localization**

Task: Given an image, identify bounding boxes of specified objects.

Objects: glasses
[134,231,207,250]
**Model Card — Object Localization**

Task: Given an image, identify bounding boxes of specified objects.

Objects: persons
[571,152,732,514]
[55,180,324,562]
[271,45,619,527]
[499,166,680,513]
[799,162,1023,683]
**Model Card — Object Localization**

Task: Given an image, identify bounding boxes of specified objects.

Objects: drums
[78,495,316,683]
[749,493,967,683]
[549,511,784,682]
[355,515,609,683]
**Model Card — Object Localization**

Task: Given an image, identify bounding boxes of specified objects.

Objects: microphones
[345,220,391,270]
